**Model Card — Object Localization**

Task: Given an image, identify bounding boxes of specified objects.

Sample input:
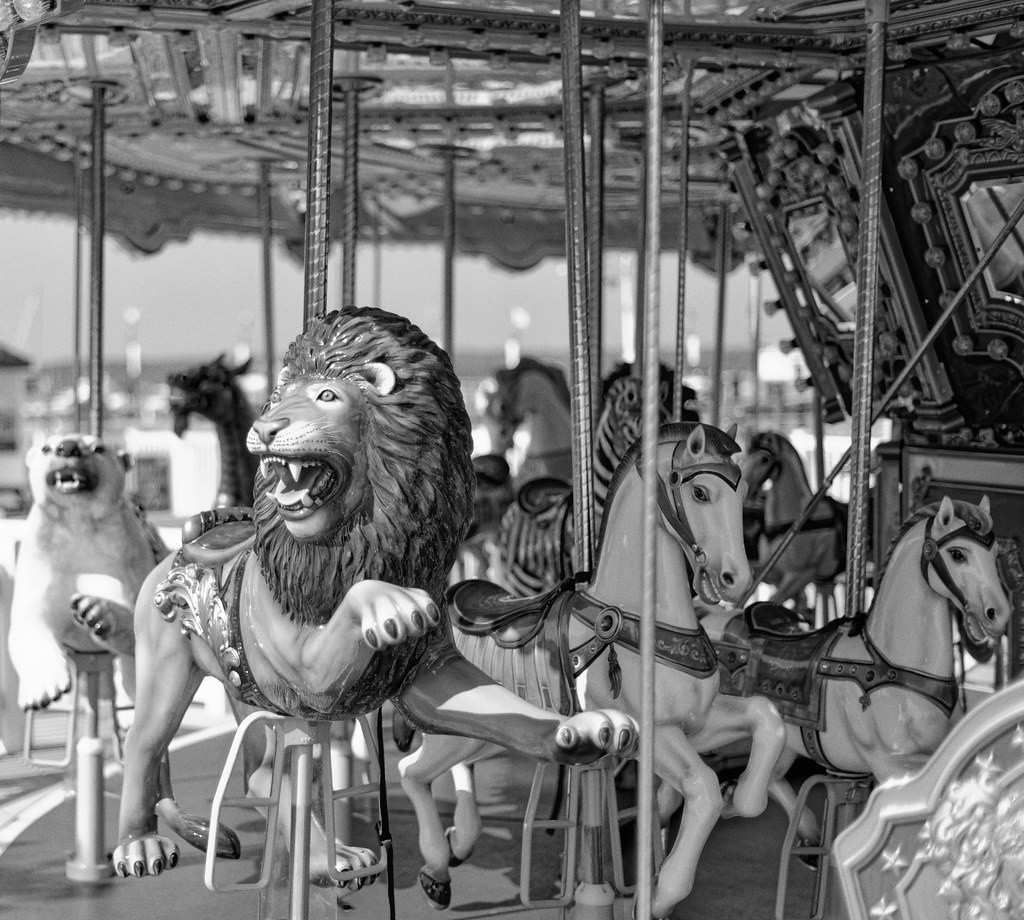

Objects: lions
[114,306,640,879]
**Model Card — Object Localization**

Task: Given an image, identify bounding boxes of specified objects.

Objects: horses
[396,356,1014,920]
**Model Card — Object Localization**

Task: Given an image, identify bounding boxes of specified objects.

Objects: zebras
[494,377,672,599]
